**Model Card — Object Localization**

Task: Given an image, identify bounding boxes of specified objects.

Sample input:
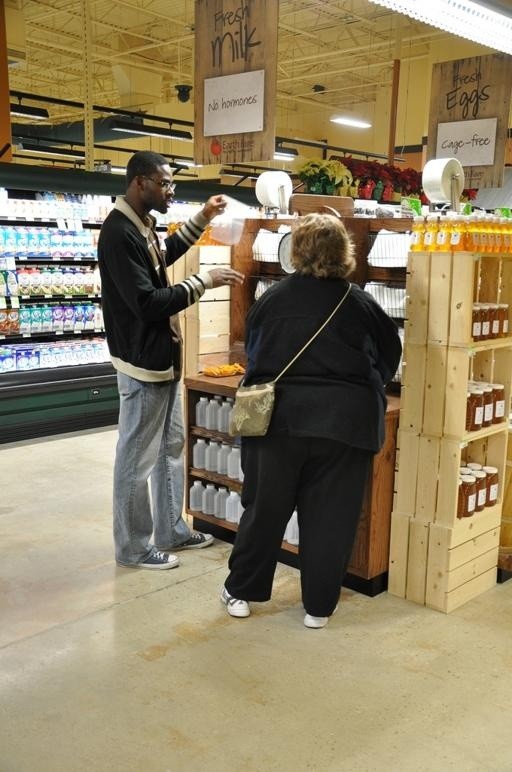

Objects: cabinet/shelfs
[388,252,512,613]
[183,219,414,597]
[0,161,221,443]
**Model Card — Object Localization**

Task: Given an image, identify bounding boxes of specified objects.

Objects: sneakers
[172,533,214,551]
[135,551,180,569]
[304,603,338,628]
[220,583,250,618]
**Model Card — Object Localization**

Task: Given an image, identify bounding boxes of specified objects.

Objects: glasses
[144,175,177,191]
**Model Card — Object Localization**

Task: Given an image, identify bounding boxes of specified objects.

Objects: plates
[277,231,297,275]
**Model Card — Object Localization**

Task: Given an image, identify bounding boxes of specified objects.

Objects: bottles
[409,211,512,254]
[188,392,304,550]
[454,300,512,518]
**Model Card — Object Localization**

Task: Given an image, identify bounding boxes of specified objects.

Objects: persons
[96,149,246,570]
[219,211,402,629]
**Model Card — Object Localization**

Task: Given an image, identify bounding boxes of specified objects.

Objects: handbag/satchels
[228,381,276,437]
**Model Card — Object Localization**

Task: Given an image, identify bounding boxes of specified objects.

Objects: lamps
[109,122,192,142]
[10,103,50,120]
[275,146,298,161]
[219,168,260,180]
[17,143,86,159]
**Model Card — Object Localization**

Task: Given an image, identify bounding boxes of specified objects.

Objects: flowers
[299,154,430,205]
[462,186,478,201]
